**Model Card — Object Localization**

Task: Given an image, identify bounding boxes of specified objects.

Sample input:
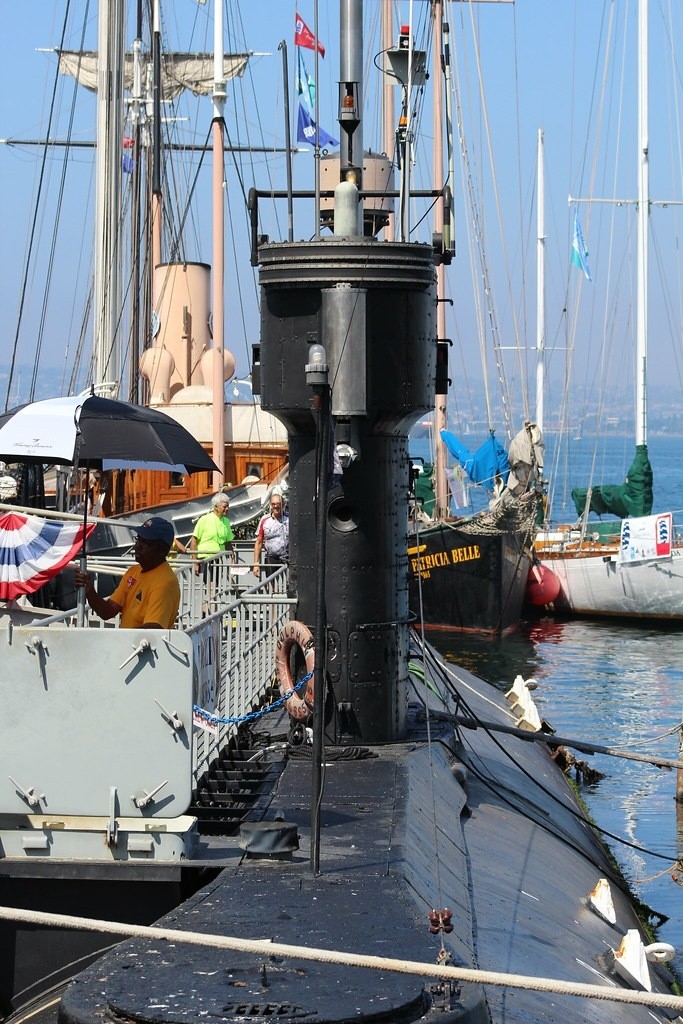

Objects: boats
[533,0,683,619]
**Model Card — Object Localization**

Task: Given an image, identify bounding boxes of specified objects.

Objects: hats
[129,517,175,549]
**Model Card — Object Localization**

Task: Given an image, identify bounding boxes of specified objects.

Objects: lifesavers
[273,620,323,721]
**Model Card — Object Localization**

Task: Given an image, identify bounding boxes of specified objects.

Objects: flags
[296,101,340,149]
[294,12,325,59]
[297,46,315,108]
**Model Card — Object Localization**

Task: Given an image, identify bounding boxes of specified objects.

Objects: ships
[3,0,683,1024]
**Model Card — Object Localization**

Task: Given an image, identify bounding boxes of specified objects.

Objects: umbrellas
[0,383,224,628]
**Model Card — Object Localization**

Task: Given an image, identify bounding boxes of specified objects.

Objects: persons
[74,517,181,630]
[253,495,290,594]
[190,493,236,619]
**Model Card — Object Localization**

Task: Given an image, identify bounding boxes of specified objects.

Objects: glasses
[131,536,156,548]
[271,503,282,507]
